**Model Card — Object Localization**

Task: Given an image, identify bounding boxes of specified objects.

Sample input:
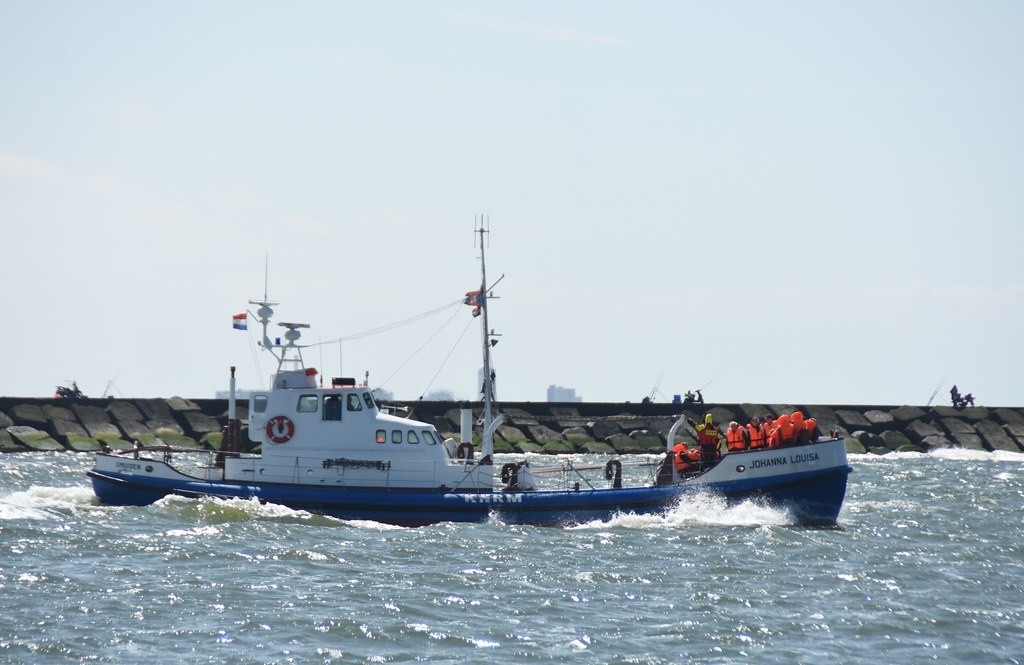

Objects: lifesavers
[266,414,296,445]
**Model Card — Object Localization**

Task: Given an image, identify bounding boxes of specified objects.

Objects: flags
[233,313,247,330]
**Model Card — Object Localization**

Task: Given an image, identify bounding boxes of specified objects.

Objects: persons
[726,411,818,452]
[950,385,957,408]
[325,395,354,420]
[673,414,721,478]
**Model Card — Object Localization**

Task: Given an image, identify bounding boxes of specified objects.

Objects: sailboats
[83,210,857,533]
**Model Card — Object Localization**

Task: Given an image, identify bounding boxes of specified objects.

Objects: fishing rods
[928,376,948,406]
[649,372,666,401]
